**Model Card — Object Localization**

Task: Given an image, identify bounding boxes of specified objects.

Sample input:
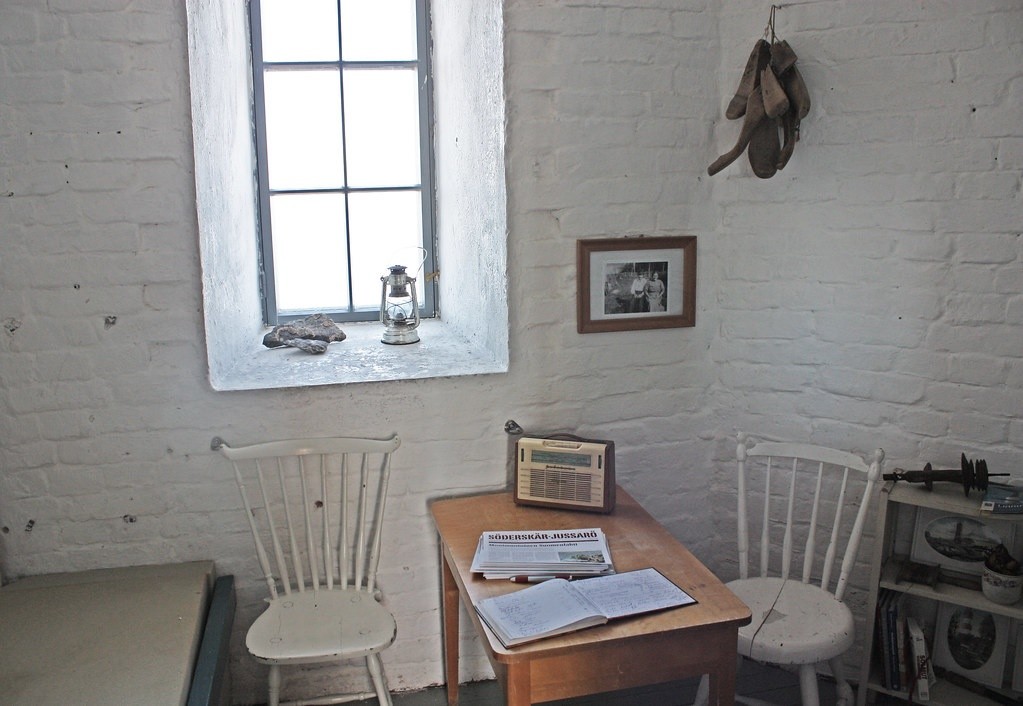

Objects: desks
[432,483,752,706]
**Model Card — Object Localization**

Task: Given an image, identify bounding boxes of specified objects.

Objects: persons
[607,271,664,312]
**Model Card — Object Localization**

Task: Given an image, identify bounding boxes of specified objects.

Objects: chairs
[693,442,885,706]
[219,435,402,706]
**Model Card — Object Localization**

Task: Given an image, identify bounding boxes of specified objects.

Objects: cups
[982,561,1023,607]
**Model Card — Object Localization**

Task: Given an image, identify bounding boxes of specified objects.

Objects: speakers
[513,433,616,513]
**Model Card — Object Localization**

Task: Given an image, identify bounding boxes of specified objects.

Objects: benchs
[0,559,238,706]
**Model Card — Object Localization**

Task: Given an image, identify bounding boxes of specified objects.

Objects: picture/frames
[910,506,1016,577]
[931,601,1011,689]
[576,235,697,334]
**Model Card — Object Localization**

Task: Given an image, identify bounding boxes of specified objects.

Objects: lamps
[380,264,421,344]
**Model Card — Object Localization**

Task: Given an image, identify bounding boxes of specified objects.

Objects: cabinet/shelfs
[856,483,1023,706]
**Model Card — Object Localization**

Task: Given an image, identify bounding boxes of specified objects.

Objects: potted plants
[982,542,1023,605]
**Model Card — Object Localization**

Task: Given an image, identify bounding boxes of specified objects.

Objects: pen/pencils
[509,574,572,583]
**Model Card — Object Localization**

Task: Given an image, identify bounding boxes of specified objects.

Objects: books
[877,592,936,700]
[473,566,698,650]
